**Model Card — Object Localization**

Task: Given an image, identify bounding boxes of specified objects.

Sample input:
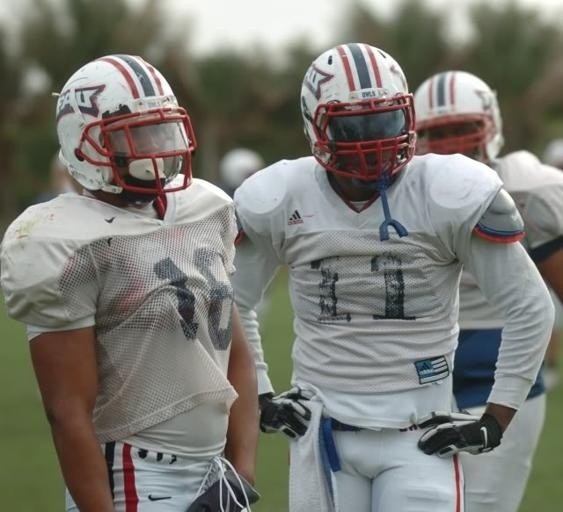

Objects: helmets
[301,43,415,181]
[413,71,505,162]
[56,54,197,194]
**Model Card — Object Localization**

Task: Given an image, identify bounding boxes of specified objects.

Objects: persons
[224,37,558,512]
[1,53,266,511]
[407,66,563,512]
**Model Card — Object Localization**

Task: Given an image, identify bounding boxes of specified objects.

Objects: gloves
[417,410,502,458]
[258,385,313,441]
[186,470,261,511]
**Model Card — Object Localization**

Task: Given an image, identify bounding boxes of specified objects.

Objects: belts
[329,416,415,431]
